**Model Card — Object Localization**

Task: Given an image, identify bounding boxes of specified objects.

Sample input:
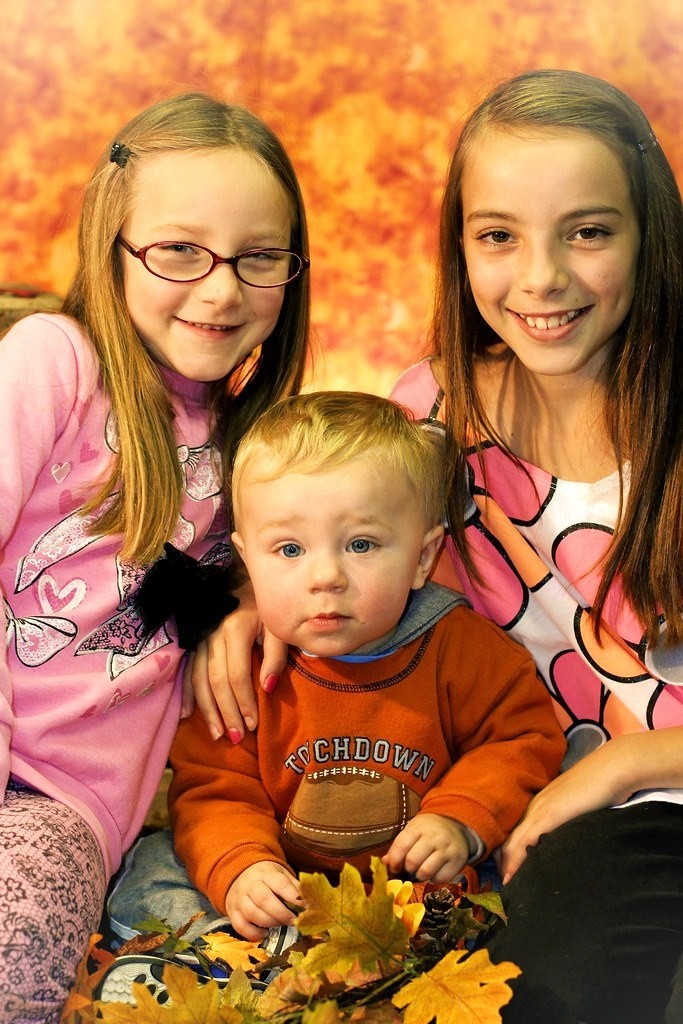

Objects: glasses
[116,234,310,288]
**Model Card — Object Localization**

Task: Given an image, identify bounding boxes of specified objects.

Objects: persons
[176,69,683,1024]
[101,388,570,1016]
[0,94,313,1024]
